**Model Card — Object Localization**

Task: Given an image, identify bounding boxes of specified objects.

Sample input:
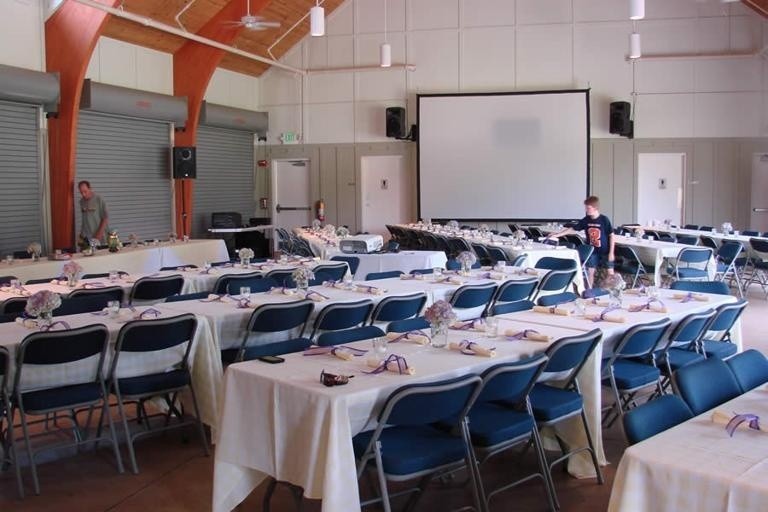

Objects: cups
[107,300,122,320]
[432,267,442,281]
[486,314,500,336]
[305,222,347,249]
[409,221,765,250]
[498,260,508,273]
[343,276,355,290]
[374,337,390,357]
[5,235,288,291]
[240,287,252,301]
[571,290,660,316]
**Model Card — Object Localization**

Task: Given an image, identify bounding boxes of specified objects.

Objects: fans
[218,1,281,36]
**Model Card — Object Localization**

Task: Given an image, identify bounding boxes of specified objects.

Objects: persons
[78,181,111,253]
[548,197,615,290]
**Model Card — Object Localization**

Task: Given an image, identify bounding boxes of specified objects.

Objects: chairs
[602,300,768,444]
[583,288,609,298]
[10,323,126,496]
[608,224,704,292]
[0,250,577,392]
[86,313,210,474]
[530,328,604,511]
[386,221,584,266]
[0,346,26,501]
[466,354,556,512]
[353,374,483,511]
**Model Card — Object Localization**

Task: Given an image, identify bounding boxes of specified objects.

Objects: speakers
[173,146,196,179]
[610,101,630,133]
[386,108,406,137]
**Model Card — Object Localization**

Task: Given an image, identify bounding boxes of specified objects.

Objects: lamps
[627,19,641,59]
[626,0,644,18]
[379,1,392,67]
[308,1,326,36]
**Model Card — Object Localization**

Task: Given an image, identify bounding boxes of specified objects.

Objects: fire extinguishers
[318,200,325,221]
